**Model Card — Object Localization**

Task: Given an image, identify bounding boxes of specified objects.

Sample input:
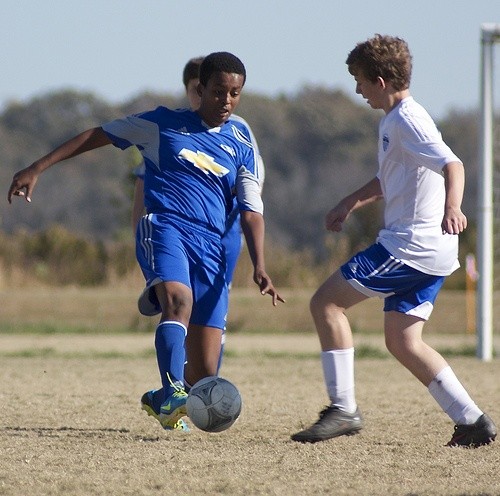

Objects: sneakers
[290,405,363,444]
[445,412,498,448]
[157,371,190,428]
[141,389,190,433]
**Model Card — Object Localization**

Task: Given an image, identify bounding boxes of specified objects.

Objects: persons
[133,56,265,377]
[292,35,497,448]
[7,51,286,431]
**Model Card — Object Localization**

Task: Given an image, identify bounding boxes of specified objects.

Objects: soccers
[185,375,242,433]
